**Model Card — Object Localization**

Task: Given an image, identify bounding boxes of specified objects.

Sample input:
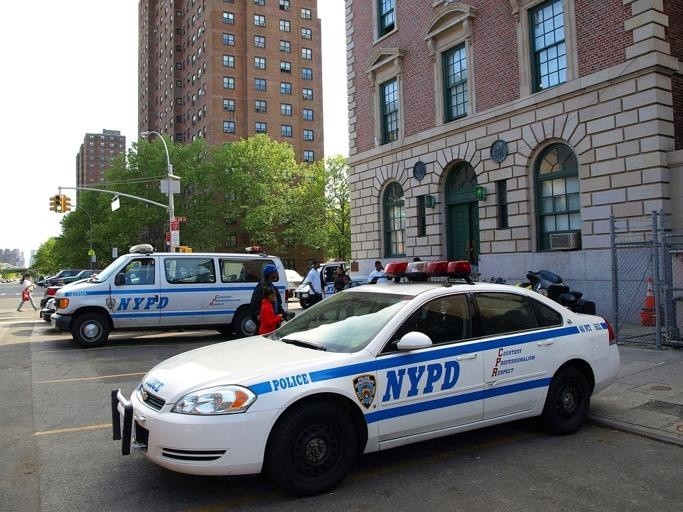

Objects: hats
[263,264,280,276]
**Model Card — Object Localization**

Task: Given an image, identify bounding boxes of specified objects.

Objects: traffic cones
[639,277,665,327]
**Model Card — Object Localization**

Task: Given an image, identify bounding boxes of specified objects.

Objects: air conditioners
[547,231,577,252]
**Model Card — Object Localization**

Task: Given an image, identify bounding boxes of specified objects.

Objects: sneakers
[17,308,22,312]
[34,307,39,311]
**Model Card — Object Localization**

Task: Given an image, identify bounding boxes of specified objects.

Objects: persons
[306,261,330,323]
[367,261,387,283]
[249,264,288,334]
[16,273,39,312]
[407,258,431,282]
[256,286,283,335]
[342,274,358,323]
[333,266,350,322]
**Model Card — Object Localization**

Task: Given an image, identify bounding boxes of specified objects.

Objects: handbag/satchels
[22,290,30,301]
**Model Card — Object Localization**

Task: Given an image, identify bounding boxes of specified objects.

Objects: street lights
[139,130,176,254]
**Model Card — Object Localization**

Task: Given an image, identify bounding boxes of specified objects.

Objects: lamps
[474,185,486,201]
[423,193,437,209]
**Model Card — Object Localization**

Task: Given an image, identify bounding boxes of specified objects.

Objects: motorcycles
[295,260,349,310]
[516,267,595,329]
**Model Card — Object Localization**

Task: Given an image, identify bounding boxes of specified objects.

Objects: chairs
[489,307,525,336]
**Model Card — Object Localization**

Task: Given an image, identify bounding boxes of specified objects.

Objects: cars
[2,277,17,283]
[35,269,104,323]
[109,259,621,500]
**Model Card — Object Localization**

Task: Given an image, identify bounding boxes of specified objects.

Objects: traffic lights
[54,194,61,212]
[60,195,71,213]
[174,246,186,253]
[49,195,56,212]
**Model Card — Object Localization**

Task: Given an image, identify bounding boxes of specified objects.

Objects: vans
[48,248,291,348]
